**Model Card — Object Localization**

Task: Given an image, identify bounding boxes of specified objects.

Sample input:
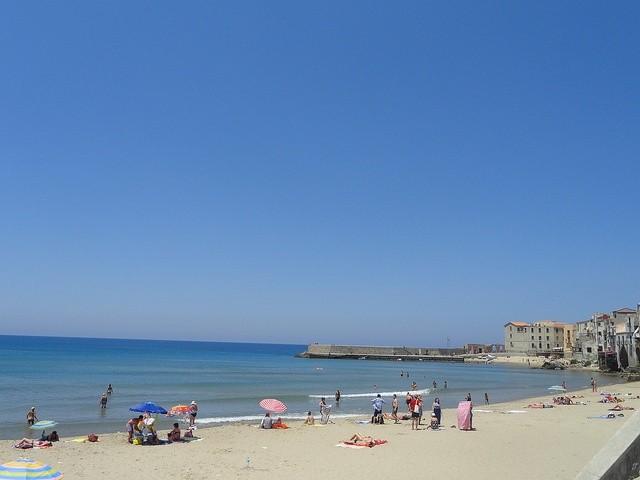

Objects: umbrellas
[128,401,168,414]
[259,399,287,413]
[29,420,58,429]
[165,403,196,416]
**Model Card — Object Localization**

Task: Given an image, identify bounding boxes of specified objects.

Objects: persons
[562,381,566,393]
[390,393,401,424]
[426,413,438,429]
[433,380,436,388]
[348,433,382,443]
[465,392,471,401]
[261,412,273,428]
[41,429,59,442]
[400,371,403,376]
[271,417,283,425]
[482,392,489,405]
[614,405,634,409]
[409,394,421,430]
[168,423,180,442]
[99,393,107,408]
[141,412,154,435]
[303,411,314,425]
[125,415,143,444]
[374,385,377,388]
[432,398,441,426]
[444,381,447,388]
[599,393,624,402]
[12,438,53,447]
[146,434,159,444]
[371,393,386,417]
[319,397,335,424]
[190,401,198,427]
[106,384,114,394]
[410,381,417,391]
[552,396,575,406]
[528,403,554,408]
[335,389,340,401]
[26,407,38,425]
[407,372,409,377]
[591,377,596,392]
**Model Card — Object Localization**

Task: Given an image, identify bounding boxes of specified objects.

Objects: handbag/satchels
[414,400,420,413]
